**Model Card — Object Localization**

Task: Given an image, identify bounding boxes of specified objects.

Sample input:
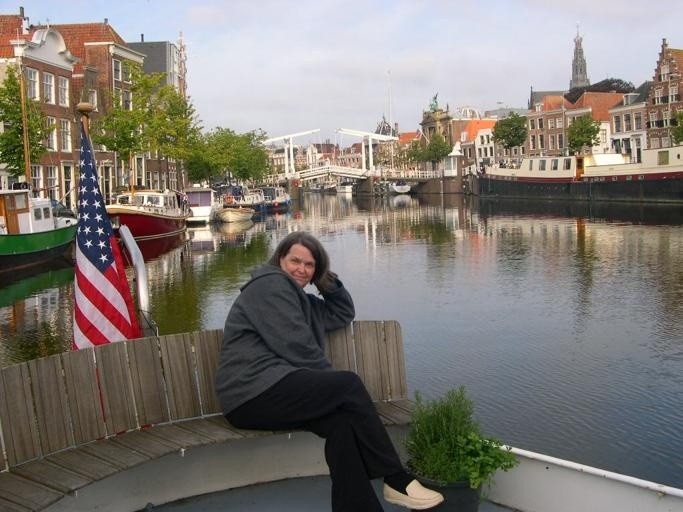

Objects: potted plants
[401,384,521,510]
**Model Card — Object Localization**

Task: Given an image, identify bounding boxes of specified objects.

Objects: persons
[214,232,445,512]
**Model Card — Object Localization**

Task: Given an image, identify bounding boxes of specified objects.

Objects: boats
[222,188,265,211]
[0,61,79,283]
[106,184,193,263]
[182,186,223,224]
[219,220,255,234]
[217,207,255,222]
[263,187,291,208]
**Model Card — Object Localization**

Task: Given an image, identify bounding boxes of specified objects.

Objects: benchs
[1,319,428,512]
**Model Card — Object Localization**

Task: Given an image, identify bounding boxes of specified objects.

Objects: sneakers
[382,478,444,509]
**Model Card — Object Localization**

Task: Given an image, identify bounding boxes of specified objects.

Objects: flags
[69,119,153,441]
